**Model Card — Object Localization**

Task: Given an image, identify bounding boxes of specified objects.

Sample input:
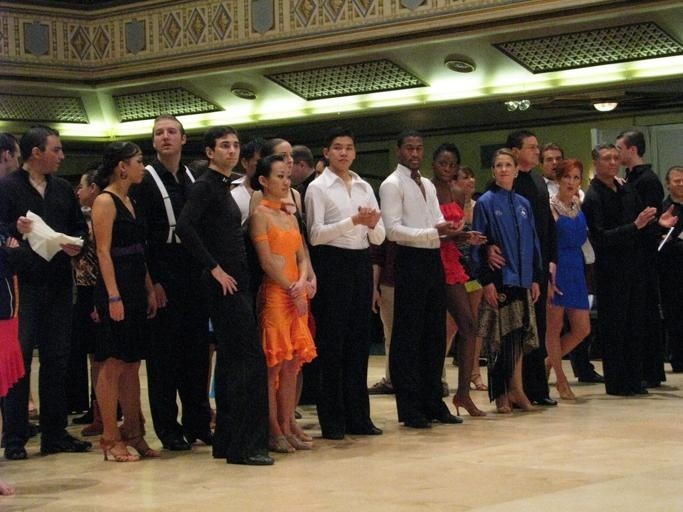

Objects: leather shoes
[226,447,275,466]
[537,398,558,405]
[368,382,394,394]
[163,439,193,452]
[211,439,230,458]
[73,408,95,424]
[185,431,214,446]
[81,420,103,437]
[3,445,28,460]
[578,372,604,383]
[437,413,463,424]
[404,418,432,428]
[40,434,93,455]
[322,430,345,440]
[364,426,383,435]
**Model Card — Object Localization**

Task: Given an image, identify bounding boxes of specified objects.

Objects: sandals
[293,422,313,442]
[269,436,296,453]
[28,398,38,419]
[284,435,311,450]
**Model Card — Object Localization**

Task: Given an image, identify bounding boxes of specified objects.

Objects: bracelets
[108,295,121,302]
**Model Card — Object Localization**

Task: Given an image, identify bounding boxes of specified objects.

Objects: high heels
[470,374,488,391]
[495,400,511,413]
[557,382,576,400]
[508,392,537,411]
[99,438,140,462]
[453,395,487,416]
[119,425,163,458]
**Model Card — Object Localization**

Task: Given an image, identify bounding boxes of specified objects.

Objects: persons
[1,131,39,495]
[545,160,590,400]
[618,131,666,388]
[663,167,683,372]
[249,138,303,234]
[485,130,558,408]
[1,126,93,460]
[250,155,316,454]
[468,149,544,413]
[129,114,215,452]
[176,126,274,466]
[92,140,158,462]
[290,146,321,204]
[539,142,605,382]
[441,166,489,397]
[230,137,267,225]
[581,142,677,397]
[72,168,122,438]
[305,129,386,441]
[379,130,467,429]
[367,241,398,394]
[430,142,487,418]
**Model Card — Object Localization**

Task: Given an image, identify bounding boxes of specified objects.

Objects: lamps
[504,99,532,113]
[593,102,619,112]
